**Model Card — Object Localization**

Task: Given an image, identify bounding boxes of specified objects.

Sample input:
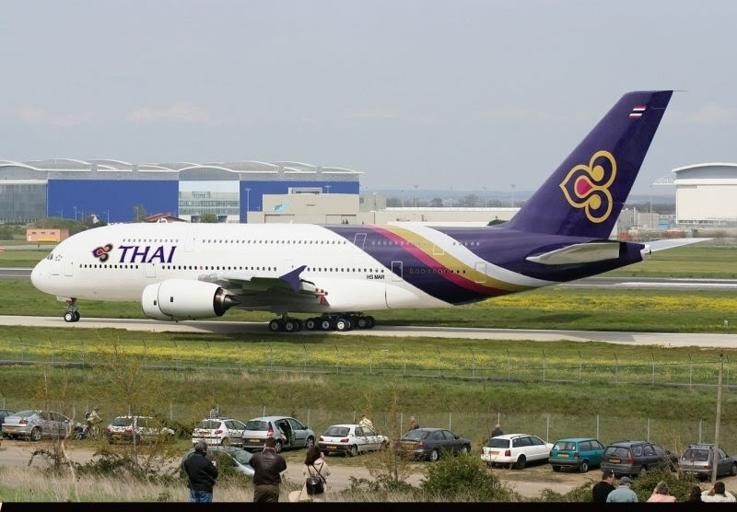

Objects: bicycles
[85,419,103,439]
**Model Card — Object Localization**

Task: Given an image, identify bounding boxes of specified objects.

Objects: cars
[318,421,390,454]
[549,436,606,472]
[107,416,176,444]
[480,433,559,468]
[1,410,88,440]
[243,416,317,451]
[191,416,246,447]
[397,426,472,462]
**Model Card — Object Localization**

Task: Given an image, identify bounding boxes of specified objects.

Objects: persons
[184,442,218,502]
[299,447,331,502]
[359,415,372,426]
[79,406,102,435]
[249,438,287,502]
[207,407,221,419]
[408,416,419,431]
[593,469,735,502]
[492,424,503,437]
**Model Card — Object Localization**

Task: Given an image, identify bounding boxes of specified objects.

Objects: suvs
[0,409,17,439]
[677,441,737,480]
[601,438,678,478]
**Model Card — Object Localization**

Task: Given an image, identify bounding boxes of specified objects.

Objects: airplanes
[25,88,673,339]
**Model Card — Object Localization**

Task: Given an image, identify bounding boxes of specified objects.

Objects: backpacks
[306,463,325,495]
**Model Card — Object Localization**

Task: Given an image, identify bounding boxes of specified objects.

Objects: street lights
[412,184,418,207]
[483,184,487,207]
[244,186,250,223]
[400,188,404,207]
[106,208,110,224]
[323,185,330,226]
[133,205,139,222]
[510,184,517,208]
[73,207,76,220]
[371,192,378,223]
[449,186,454,207]
